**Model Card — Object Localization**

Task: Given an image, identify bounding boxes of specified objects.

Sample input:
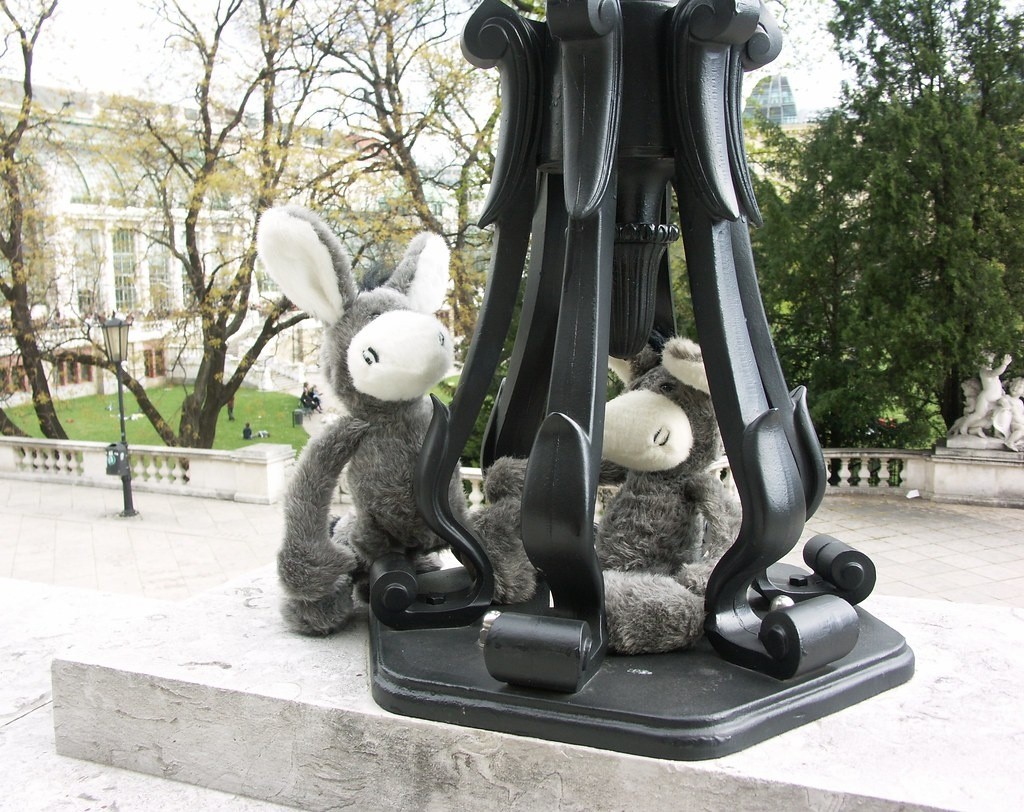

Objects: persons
[947,339,1024,453]
[243,421,270,439]
[301,381,325,413]
[228,395,236,420]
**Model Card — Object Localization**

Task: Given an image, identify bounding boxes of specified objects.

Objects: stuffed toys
[255,200,543,630]
[484,334,749,653]
